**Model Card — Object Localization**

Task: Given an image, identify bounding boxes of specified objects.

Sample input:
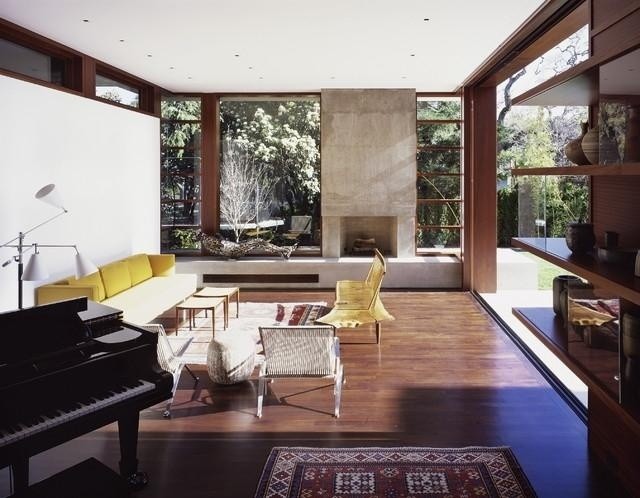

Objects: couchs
[38,251,197,327]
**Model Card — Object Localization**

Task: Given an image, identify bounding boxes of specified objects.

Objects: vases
[561,120,591,166]
[581,124,618,166]
[563,220,599,255]
[552,274,580,315]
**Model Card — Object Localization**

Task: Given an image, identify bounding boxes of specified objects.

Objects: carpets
[160,301,328,365]
[250,444,537,497]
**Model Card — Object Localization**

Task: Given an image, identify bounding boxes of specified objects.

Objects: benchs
[316,248,397,344]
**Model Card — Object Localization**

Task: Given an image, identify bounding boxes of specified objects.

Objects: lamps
[0,183,99,308]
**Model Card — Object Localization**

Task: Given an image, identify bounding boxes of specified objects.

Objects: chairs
[254,325,347,418]
[129,323,199,417]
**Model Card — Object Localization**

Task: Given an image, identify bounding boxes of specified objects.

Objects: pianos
[0,299,173,498]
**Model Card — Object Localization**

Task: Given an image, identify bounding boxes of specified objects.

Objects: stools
[5,457,138,496]
[207,332,255,384]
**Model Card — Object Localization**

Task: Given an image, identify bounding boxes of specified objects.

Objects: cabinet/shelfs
[507,37,639,435]
[415,91,464,257]
[160,95,206,256]
